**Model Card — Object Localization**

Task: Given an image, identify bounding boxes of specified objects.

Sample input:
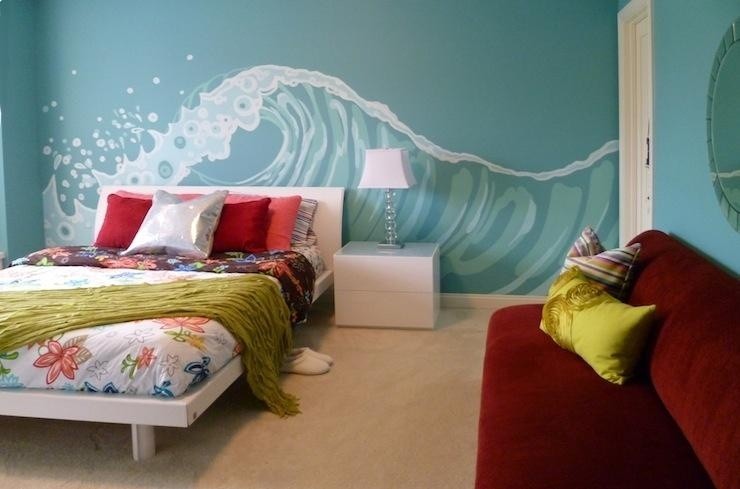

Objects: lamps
[357,144,419,249]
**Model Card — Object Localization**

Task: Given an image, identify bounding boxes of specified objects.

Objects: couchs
[470,228,740,487]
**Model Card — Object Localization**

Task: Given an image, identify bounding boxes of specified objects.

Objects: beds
[0,175,346,463]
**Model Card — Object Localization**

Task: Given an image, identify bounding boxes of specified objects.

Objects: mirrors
[702,16,740,233]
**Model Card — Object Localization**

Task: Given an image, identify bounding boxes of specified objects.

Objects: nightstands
[327,239,447,332]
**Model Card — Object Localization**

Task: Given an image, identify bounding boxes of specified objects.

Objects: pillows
[92,187,321,260]
[536,263,659,388]
[553,223,643,304]
